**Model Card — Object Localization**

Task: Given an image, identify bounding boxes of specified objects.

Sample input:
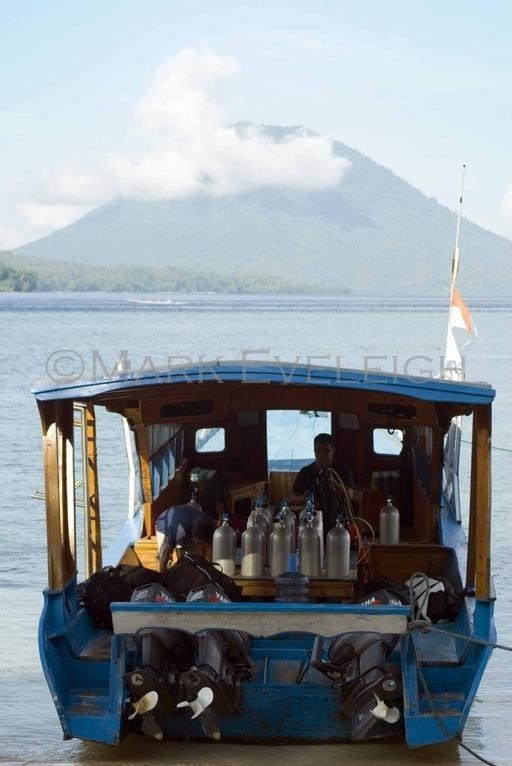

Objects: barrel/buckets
[273,555,308,604]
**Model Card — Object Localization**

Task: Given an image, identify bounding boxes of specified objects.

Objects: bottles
[211,490,400,580]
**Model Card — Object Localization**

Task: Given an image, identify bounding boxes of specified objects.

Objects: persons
[288,433,354,520]
[154,503,218,577]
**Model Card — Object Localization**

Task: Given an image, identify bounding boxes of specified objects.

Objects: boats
[29,363,500,749]
